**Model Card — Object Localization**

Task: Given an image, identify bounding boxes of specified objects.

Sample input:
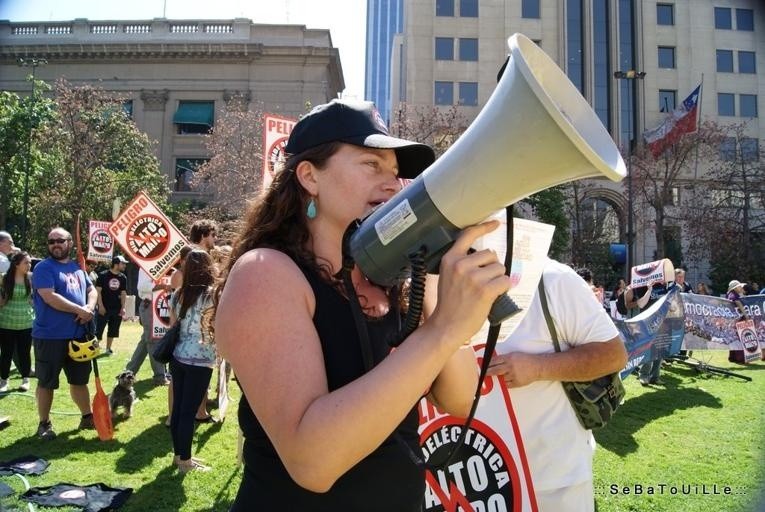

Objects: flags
[642,73,703,162]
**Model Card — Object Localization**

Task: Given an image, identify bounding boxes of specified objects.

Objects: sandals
[196,415,220,424]
[208,399,218,406]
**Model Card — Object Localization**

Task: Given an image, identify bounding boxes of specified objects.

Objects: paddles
[76,216,114,441]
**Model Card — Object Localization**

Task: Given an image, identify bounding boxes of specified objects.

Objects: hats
[112,255,129,264]
[726,280,747,294]
[285,99,437,177]
[214,244,234,257]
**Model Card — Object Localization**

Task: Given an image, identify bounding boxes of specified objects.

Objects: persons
[170,219,233,472]
[696,282,709,295]
[0,251,34,392]
[728,280,749,365]
[0,232,36,377]
[126,266,170,385]
[623,284,641,373]
[576,266,592,287]
[751,281,758,293]
[417,255,628,512]
[85,259,98,284]
[674,268,695,355]
[215,100,512,512]
[31,228,98,440]
[609,280,625,302]
[96,255,129,355]
[637,279,668,386]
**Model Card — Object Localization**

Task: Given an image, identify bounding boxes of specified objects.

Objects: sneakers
[78,416,96,430]
[178,463,211,473]
[173,455,204,465]
[0,378,7,391]
[638,373,649,385]
[30,368,35,376]
[37,423,56,440]
[154,376,170,385]
[106,347,114,354]
[20,378,30,391]
[655,379,666,385]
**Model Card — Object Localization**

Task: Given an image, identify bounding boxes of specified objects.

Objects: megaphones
[340,33,629,350]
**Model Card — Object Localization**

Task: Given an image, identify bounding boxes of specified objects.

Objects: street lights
[614,69,646,286]
[16,57,48,248]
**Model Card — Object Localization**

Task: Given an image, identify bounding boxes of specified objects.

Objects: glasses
[88,264,97,268]
[48,238,70,244]
[209,232,217,236]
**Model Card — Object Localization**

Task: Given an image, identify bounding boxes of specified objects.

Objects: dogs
[110,370,136,418]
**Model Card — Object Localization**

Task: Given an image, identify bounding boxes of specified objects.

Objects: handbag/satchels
[538,274,626,430]
[153,289,206,365]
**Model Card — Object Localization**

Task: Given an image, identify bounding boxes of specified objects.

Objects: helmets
[68,333,99,362]
